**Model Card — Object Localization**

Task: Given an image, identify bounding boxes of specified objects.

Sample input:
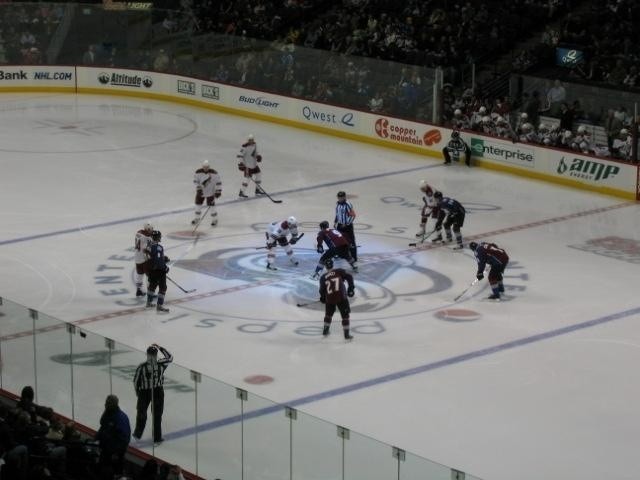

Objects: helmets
[144,223,161,241]
[248,135,254,141]
[470,242,478,250]
[201,160,210,167]
[287,217,297,226]
[320,221,329,228]
[452,107,589,150]
[620,129,629,135]
[337,191,346,197]
[325,259,333,269]
[147,347,157,354]
[420,181,442,199]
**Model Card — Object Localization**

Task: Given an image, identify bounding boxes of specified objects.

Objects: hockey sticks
[190,204,210,235]
[251,177,282,203]
[418,229,424,249]
[297,292,353,307]
[165,275,196,294]
[408,228,436,246]
[256,232,304,250]
[454,279,480,302]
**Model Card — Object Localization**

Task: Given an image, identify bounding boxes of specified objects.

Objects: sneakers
[132,433,140,439]
[322,328,351,338]
[416,230,463,250]
[154,438,164,442]
[192,217,218,225]
[267,257,299,271]
[488,285,504,299]
[137,287,169,311]
[238,187,267,198]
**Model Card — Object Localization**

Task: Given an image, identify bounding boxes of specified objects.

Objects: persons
[134,223,155,296]
[415,178,446,243]
[469,238,510,300]
[2,1,639,169]
[264,216,299,271]
[333,190,357,263]
[144,229,171,313]
[191,156,222,229]
[0,384,184,479]
[318,258,355,341]
[238,136,265,202]
[310,219,359,280]
[131,341,174,448]
[433,189,466,250]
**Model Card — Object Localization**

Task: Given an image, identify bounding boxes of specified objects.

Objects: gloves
[256,155,261,162]
[347,288,354,297]
[319,295,326,303]
[290,238,296,244]
[266,243,274,249]
[316,246,324,254]
[239,163,245,171]
[215,192,221,198]
[477,271,483,279]
